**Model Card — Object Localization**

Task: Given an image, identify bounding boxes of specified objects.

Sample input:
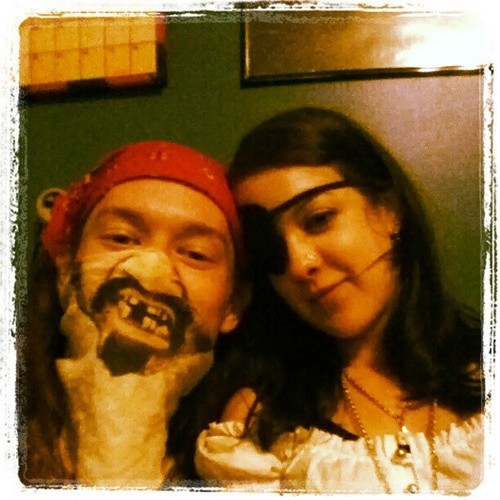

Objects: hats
[39,141,247,281]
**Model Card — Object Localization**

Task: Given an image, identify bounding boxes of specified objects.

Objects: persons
[26,139,248,492]
[167,104,483,495]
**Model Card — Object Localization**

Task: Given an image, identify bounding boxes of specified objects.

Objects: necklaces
[339,356,442,494]
